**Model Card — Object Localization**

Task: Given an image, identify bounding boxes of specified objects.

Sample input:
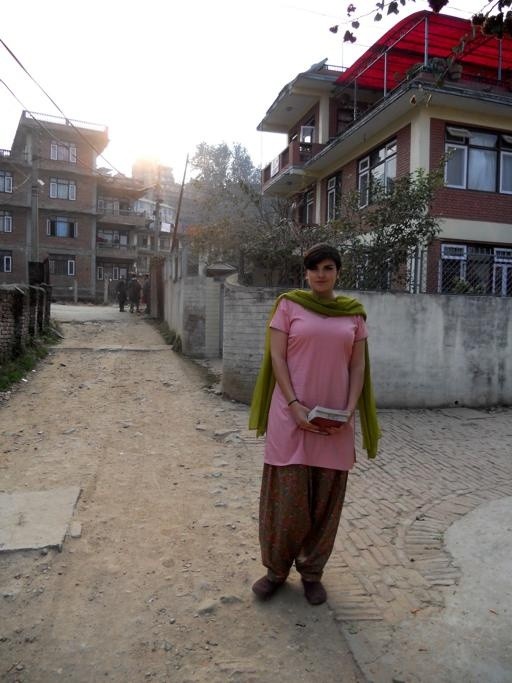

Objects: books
[303,406,352,436]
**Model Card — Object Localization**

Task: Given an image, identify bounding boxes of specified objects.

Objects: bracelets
[288,399,299,407]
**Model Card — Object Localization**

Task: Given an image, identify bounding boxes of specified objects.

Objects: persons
[126,272,142,313]
[252,242,369,605]
[116,275,126,312]
[142,273,150,312]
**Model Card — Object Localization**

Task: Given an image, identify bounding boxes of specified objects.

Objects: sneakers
[301,577,326,604]
[251,576,284,601]
